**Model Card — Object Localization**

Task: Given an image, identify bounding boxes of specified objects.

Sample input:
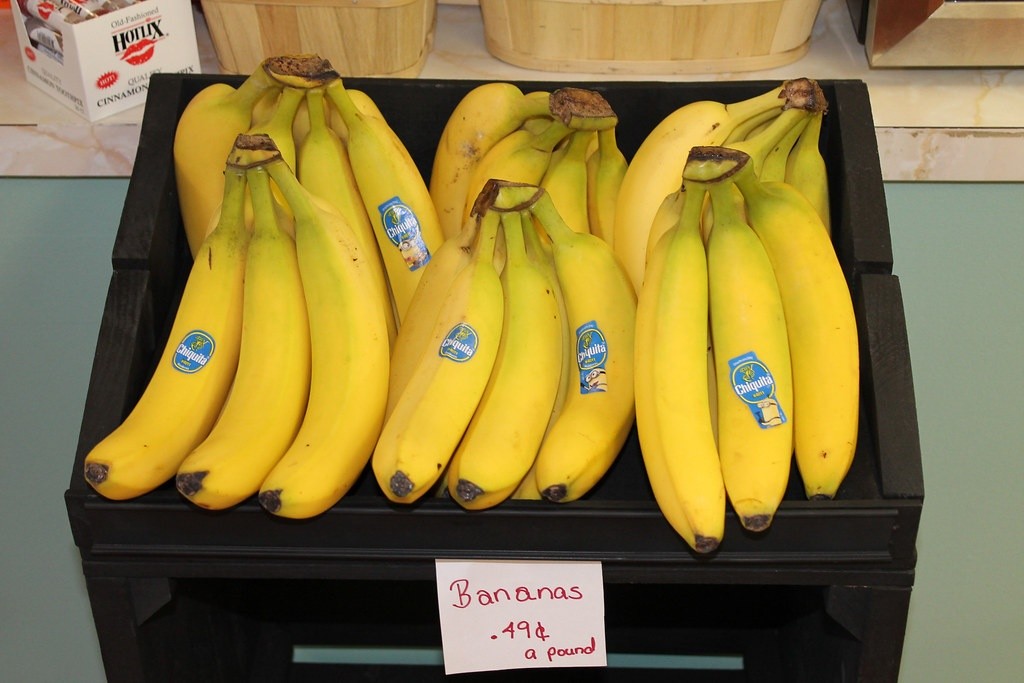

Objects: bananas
[81,54,859,555]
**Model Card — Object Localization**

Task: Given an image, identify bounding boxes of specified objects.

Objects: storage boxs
[9,0,202,124]
[62,72,930,683]
[478,0,828,77]
[201,0,440,79]
